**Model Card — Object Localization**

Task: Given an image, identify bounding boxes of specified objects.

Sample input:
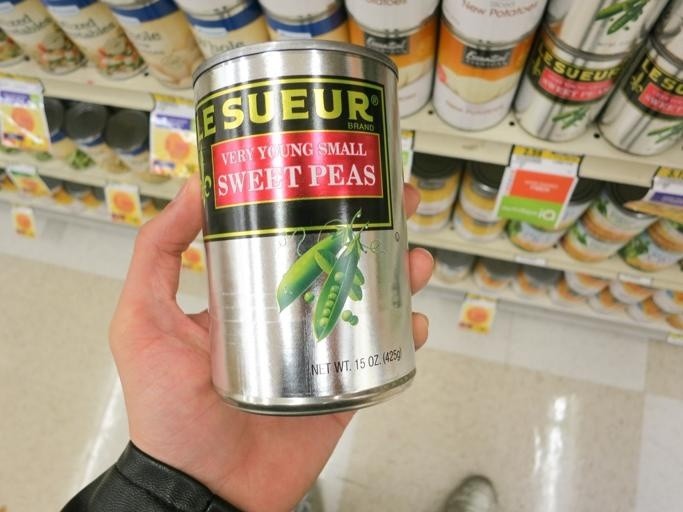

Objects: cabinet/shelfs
[1,60,683,346]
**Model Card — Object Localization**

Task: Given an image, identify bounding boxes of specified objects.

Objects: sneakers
[444,475,498,512]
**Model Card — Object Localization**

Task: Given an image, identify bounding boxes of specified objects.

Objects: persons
[59,172,500,512]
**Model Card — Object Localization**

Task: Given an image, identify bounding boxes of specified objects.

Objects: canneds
[1,1,683,158]
[1,95,683,329]
[193,39,417,414]
